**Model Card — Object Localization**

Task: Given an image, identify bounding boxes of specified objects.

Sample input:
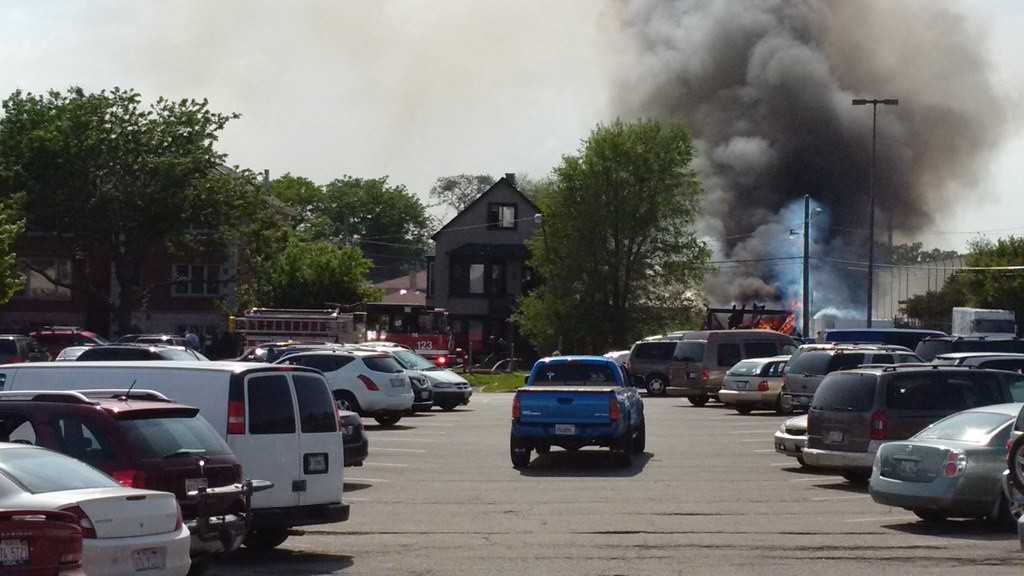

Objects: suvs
[277,345,434,416]
[0,326,210,361]
[0,379,274,575]
[1003,399,1024,549]
[232,340,415,425]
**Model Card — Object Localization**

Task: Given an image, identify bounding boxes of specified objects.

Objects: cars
[1,439,191,576]
[774,414,807,467]
[781,328,1024,412]
[339,408,369,468]
[665,330,817,406]
[603,332,682,396]
[868,401,1024,521]
[719,355,792,414]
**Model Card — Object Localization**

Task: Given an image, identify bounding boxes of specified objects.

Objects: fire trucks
[228,303,471,368]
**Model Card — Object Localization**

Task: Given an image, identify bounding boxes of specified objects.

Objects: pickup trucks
[508,356,645,467]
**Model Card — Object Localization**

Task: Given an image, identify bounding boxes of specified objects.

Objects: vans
[375,347,474,410]
[802,362,1024,481]
[0,361,350,548]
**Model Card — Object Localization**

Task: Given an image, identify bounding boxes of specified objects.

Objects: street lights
[789,99,899,335]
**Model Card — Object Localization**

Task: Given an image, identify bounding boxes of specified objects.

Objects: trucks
[952,306,1017,340]
